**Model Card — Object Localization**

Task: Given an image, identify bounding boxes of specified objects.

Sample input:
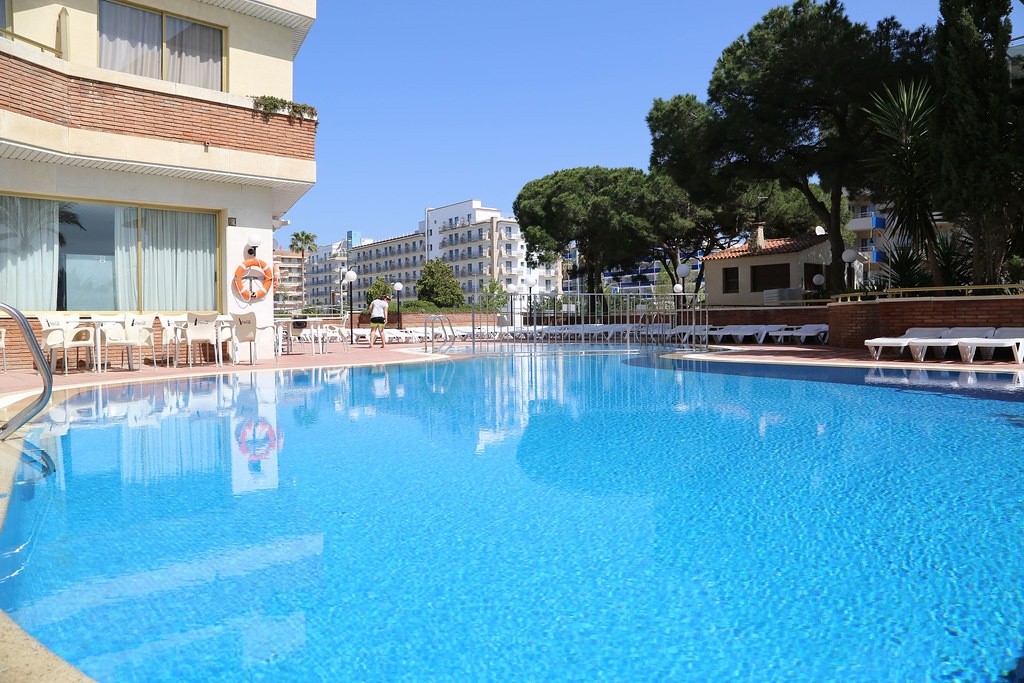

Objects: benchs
[763,289,785,305]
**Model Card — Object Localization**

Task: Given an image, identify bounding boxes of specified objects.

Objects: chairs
[0,329,7,373]
[958,327,1024,365]
[865,325,950,361]
[35,308,352,380]
[910,326,995,362]
[353,323,828,345]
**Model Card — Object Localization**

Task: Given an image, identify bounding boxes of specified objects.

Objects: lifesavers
[273,263,279,294]
[237,420,276,460]
[234,258,272,300]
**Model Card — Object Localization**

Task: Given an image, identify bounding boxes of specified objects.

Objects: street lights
[507,284,516,327]
[524,275,536,326]
[394,281,404,330]
[677,263,695,310]
[345,271,359,344]
[673,284,683,326]
[843,248,857,294]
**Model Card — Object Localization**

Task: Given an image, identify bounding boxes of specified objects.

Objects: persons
[368,295,388,349]
[371,366,390,399]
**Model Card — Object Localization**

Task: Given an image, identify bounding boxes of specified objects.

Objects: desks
[276,317,323,358]
[50,319,145,374]
[174,318,239,366]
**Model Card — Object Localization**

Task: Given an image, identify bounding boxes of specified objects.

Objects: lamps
[812,274,826,286]
[841,248,858,263]
[346,270,357,281]
[394,281,404,290]
[677,264,690,277]
[674,284,683,293]
[244,234,267,259]
